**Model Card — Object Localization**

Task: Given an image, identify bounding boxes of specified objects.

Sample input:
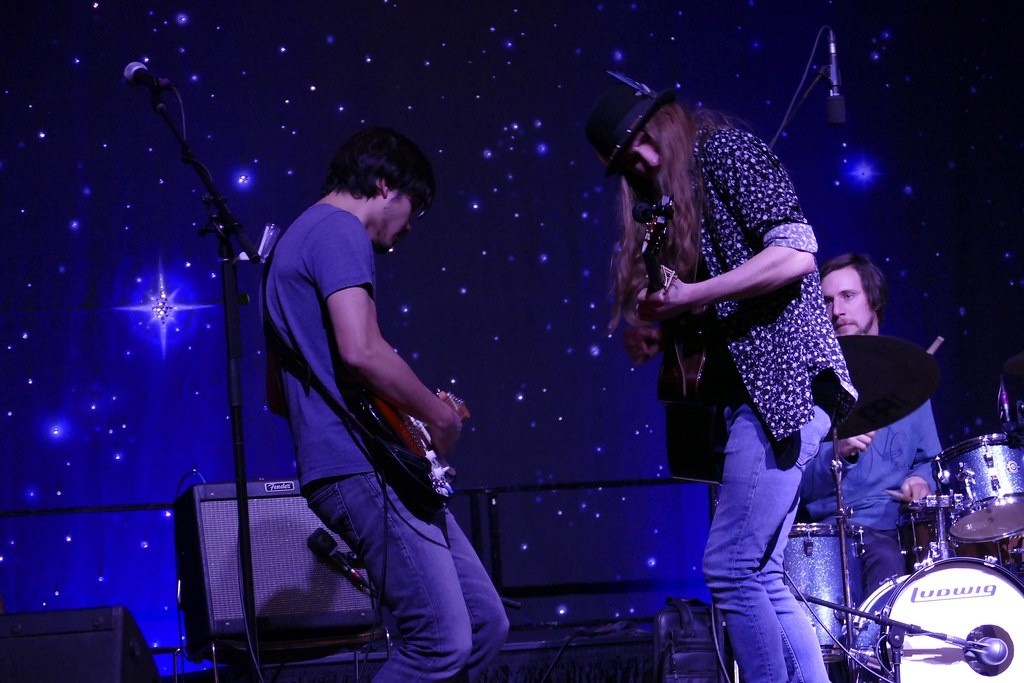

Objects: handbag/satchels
[653,596,718,683]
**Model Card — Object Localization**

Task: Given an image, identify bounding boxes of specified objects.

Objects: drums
[932,433,1024,542]
[844,553,1024,681]
[783,522,867,660]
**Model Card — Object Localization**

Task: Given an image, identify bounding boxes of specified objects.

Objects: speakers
[177,478,382,652]
[0,605,167,683]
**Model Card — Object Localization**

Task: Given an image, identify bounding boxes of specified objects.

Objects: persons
[585,69,861,683]
[783,250,942,603]
[262,128,511,683]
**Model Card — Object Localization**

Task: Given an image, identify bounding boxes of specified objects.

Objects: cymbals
[819,334,937,440]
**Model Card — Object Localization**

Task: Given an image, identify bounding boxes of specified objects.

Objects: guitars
[356,385,471,517]
[638,196,726,488]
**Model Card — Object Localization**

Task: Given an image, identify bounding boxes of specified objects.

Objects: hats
[584,70,675,176]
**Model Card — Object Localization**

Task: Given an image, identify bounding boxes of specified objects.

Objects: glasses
[402,189,426,217]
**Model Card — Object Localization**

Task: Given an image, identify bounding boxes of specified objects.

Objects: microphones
[307,527,371,588]
[123,60,175,91]
[825,30,847,124]
